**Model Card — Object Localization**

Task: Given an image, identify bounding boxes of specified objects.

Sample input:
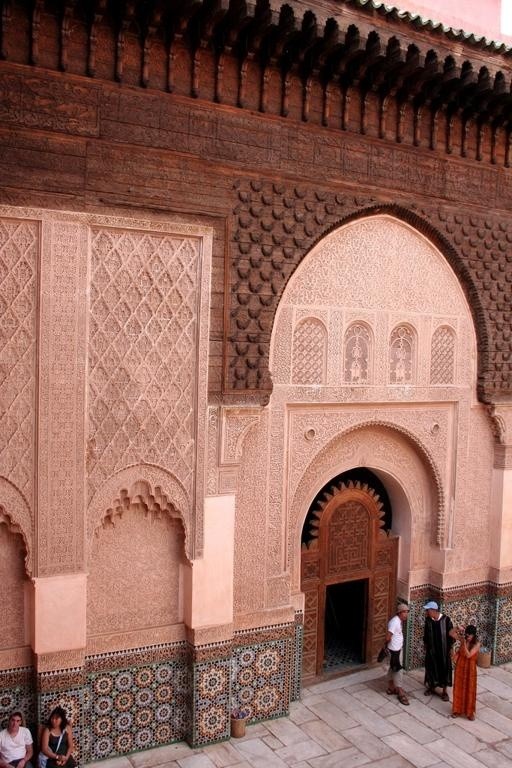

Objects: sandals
[386,687,475,721]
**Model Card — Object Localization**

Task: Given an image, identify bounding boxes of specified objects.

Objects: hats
[395,604,410,615]
[422,601,438,610]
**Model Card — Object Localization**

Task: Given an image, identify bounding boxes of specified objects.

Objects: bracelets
[56,754,59,760]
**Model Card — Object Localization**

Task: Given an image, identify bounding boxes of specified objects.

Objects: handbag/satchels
[38,746,52,768]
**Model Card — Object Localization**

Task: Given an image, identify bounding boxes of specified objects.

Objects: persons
[447,624,481,720]
[381,603,409,706]
[37,707,76,768]
[0,712,35,768]
[422,600,456,701]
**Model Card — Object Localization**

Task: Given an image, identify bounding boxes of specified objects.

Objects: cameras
[456,632,465,640]
